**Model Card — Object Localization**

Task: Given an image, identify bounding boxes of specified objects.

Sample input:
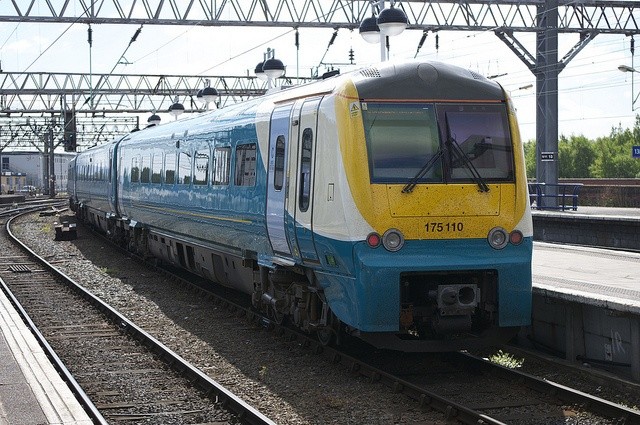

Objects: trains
[66,60,535,347]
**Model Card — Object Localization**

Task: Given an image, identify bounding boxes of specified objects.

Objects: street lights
[356,1,411,65]
[617,62,639,74]
[253,45,293,94]
[194,77,219,114]
[165,95,185,119]
[147,107,163,129]
